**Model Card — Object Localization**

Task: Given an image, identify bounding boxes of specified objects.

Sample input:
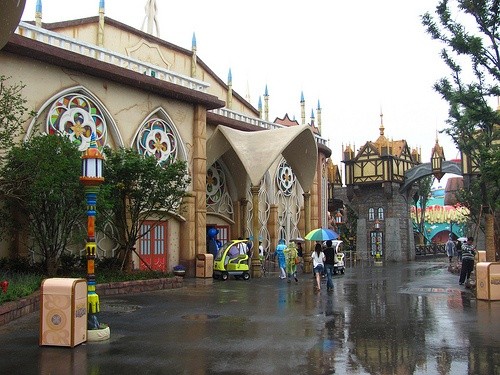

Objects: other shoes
[294,277,298,282]
[287,280,291,283]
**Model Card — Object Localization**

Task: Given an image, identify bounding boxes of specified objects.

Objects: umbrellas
[289,238,305,244]
[304,226,339,252]
[457,238,468,243]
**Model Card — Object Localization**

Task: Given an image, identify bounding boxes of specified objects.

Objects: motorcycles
[213,239,255,281]
[320,240,346,275]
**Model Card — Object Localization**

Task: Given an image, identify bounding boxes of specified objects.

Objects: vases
[173,271,185,276]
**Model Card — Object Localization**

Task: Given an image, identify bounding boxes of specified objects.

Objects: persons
[273,239,287,279]
[445,237,455,260]
[456,241,466,260]
[309,244,326,290]
[284,242,298,283]
[246,238,252,256]
[323,240,333,291]
[259,242,263,256]
[216,240,222,248]
[459,237,476,285]
[298,242,302,257]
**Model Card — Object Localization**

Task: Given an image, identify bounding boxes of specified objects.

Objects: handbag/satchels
[333,247,338,263]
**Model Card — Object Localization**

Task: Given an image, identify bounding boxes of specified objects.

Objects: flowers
[174,264,186,271]
